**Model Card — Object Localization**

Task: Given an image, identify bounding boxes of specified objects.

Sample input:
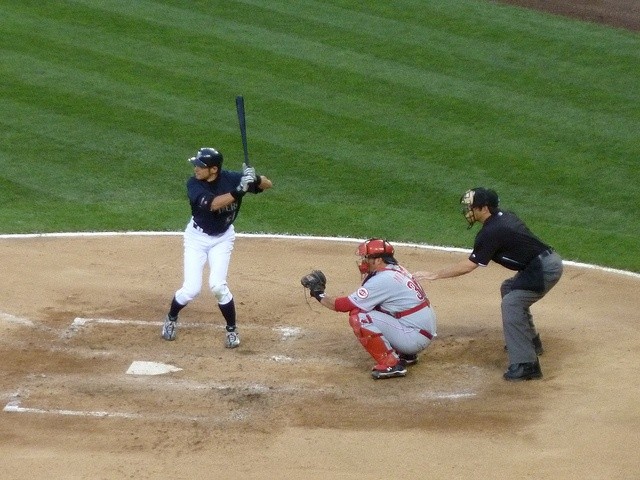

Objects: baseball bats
[236,96,254,185]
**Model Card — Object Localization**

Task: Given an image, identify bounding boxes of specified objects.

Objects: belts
[419,329,433,339]
[193,222,224,237]
[538,248,555,259]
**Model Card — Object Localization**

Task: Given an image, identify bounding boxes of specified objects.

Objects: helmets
[188,147,223,169]
[461,186,499,230]
[355,238,395,274]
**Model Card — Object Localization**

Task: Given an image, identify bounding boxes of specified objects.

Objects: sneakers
[399,352,419,365]
[372,364,407,379]
[225,323,240,349]
[162,314,178,341]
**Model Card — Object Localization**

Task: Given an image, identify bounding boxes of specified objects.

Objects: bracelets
[314,292,326,301]
[229,184,245,199]
[254,175,262,186]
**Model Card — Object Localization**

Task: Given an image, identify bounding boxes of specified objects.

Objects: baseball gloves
[300,269,327,303]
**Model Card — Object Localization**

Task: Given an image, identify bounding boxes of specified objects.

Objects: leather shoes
[504,334,543,356]
[503,362,543,381]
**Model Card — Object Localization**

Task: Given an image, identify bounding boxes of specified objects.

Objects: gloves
[229,176,255,201]
[242,163,262,184]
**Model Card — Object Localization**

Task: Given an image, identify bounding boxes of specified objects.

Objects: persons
[415,187,562,381]
[162,147,273,348]
[301,238,437,380]
